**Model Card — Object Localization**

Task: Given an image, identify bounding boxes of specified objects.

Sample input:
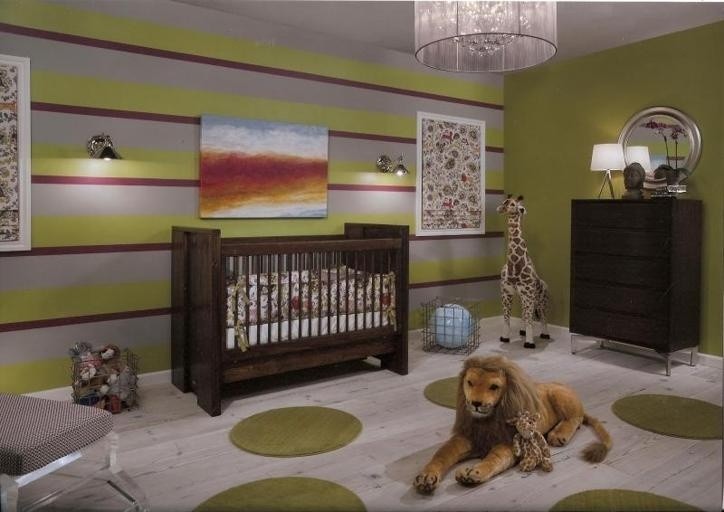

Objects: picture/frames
[414,111,486,237]
[0,54,31,253]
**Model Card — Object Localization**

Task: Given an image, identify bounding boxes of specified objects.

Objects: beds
[168,222,409,416]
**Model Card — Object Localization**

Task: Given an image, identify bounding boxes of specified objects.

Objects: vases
[654,165,674,181]
[664,170,688,185]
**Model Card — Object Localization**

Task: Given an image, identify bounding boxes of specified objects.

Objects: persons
[621,162,647,200]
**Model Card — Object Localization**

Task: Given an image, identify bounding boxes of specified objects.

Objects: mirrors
[616,107,702,190]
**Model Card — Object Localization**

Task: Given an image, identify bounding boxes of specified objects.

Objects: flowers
[666,123,687,170]
[639,121,670,166]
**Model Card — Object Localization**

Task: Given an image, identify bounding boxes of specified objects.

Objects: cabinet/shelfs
[569,199,700,376]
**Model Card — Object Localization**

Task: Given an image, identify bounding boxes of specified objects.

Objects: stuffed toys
[77,343,131,408]
[505,408,553,473]
[495,193,551,349]
[411,351,612,494]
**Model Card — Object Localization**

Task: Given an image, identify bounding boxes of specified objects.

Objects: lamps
[590,143,626,200]
[85,133,124,161]
[375,152,409,177]
[623,146,653,176]
[413,1,558,74]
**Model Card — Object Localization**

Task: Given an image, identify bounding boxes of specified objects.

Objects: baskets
[71,348,140,414]
[421,296,481,355]
